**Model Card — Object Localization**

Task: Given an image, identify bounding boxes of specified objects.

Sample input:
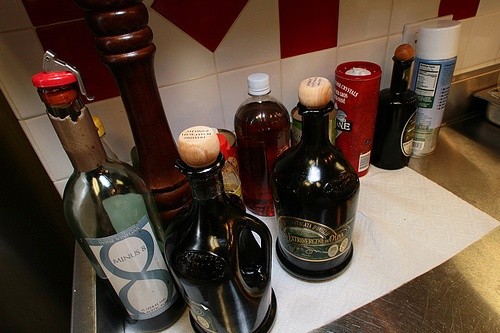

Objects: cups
[216,129,244,202]
[290,105,335,146]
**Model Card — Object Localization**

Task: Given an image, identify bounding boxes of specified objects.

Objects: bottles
[234,73,290,217]
[274,77,360,283]
[164,126,278,333]
[369,44,418,170]
[31,69,186,333]
